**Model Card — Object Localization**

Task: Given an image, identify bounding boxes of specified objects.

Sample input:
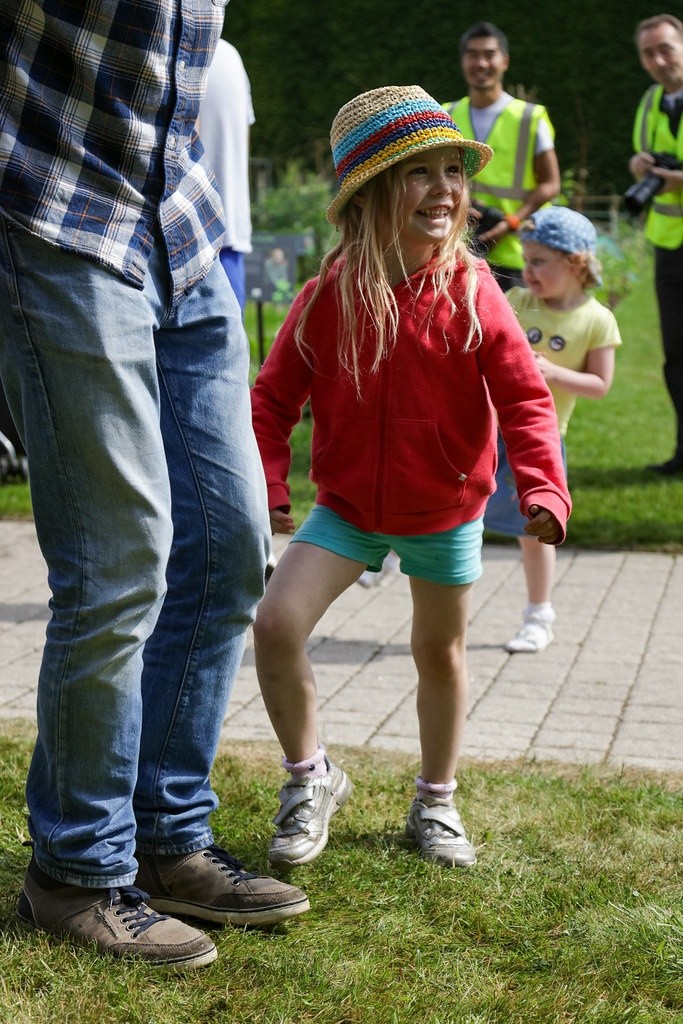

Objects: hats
[327,84,494,224]
[521,207,605,288]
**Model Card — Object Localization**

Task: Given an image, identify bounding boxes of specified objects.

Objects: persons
[0,0,310,974]
[357,23,623,653]
[250,85,575,868]
[630,14,683,477]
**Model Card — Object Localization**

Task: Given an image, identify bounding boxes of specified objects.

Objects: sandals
[358,551,396,588]
[506,603,553,654]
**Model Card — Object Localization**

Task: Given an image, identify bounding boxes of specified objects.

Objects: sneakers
[266,757,352,865]
[406,794,478,867]
[15,843,311,973]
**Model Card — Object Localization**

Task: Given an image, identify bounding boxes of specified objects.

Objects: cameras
[470,203,503,253]
[622,150,683,217]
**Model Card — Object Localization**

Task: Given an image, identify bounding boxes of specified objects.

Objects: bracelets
[504,214,520,230]
